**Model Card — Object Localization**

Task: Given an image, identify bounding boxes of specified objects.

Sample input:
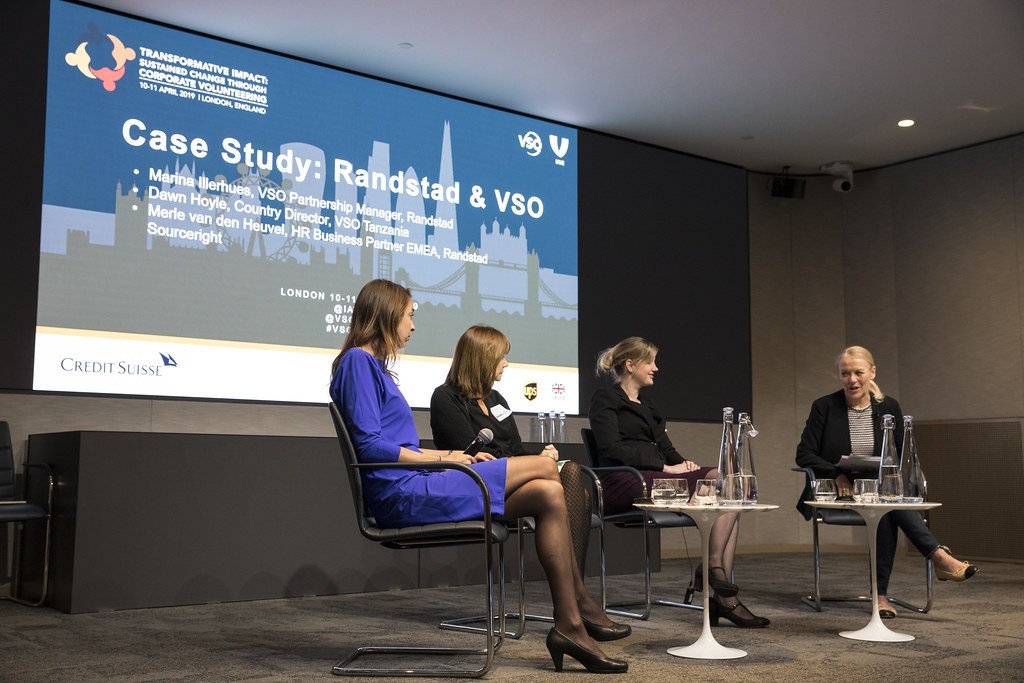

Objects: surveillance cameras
[832,179,852,192]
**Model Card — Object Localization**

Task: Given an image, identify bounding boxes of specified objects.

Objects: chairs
[0,421,53,607]
[790,467,933,613]
[577,428,735,620]
[327,401,509,676]
[437,465,606,641]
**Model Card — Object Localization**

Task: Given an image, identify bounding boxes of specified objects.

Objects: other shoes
[934,560,979,581]
[878,604,897,618]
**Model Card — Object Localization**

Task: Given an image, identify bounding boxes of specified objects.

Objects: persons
[431,326,594,580]
[331,279,632,673]
[586,336,772,628]
[796,345,980,618]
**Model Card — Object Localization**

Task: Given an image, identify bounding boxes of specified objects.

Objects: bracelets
[436,456,441,462]
[447,449,454,455]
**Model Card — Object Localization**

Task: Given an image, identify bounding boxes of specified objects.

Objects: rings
[842,487,846,493]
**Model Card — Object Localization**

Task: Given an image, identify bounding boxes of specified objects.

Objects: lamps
[767,165,806,201]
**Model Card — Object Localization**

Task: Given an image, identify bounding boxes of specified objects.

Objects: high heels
[581,615,632,641]
[709,596,771,628]
[693,563,739,598]
[546,626,629,672]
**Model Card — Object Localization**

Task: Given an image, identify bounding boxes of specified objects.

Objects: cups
[814,479,837,502]
[695,479,717,505]
[650,478,690,506]
[852,479,879,503]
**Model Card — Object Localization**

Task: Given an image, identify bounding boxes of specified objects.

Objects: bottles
[538,412,545,443]
[549,411,556,443]
[559,411,566,443]
[879,414,924,503]
[716,407,758,506]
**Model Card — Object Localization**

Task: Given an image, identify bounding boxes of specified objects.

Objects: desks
[19,429,661,614]
[805,500,942,642]
[633,501,778,659]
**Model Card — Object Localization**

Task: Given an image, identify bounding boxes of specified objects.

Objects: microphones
[876,411,881,417]
[462,429,494,456]
